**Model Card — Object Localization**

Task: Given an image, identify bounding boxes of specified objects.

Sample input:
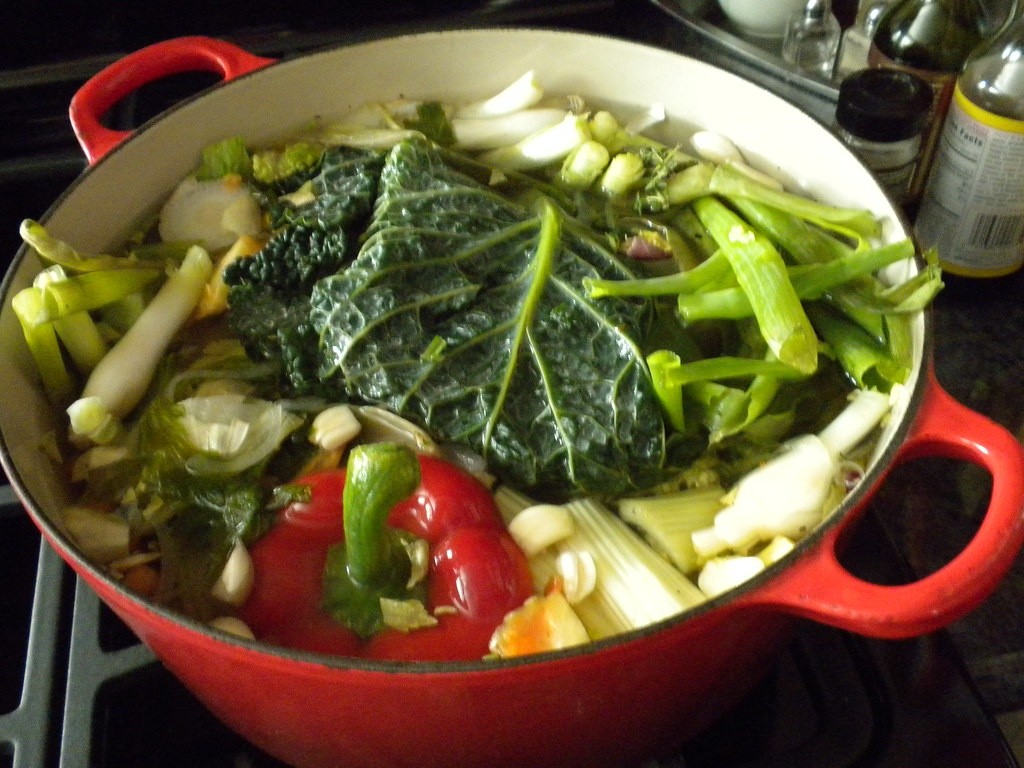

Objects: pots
[0,28,1024,766]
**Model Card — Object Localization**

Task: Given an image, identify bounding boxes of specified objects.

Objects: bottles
[910,0,1024,300]
[864,0,978,215]
[835,3,889,74]
[782,0,841,77]
[831,67,933,206]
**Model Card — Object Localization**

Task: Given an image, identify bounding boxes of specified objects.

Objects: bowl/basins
[715,0,807,41]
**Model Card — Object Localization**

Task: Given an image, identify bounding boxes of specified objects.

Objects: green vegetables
[9,67,947,654]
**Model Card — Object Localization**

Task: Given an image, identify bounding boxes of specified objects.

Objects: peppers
[239,441,535,667]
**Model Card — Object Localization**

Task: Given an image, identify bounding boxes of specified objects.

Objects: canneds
[834,66,934,206]
